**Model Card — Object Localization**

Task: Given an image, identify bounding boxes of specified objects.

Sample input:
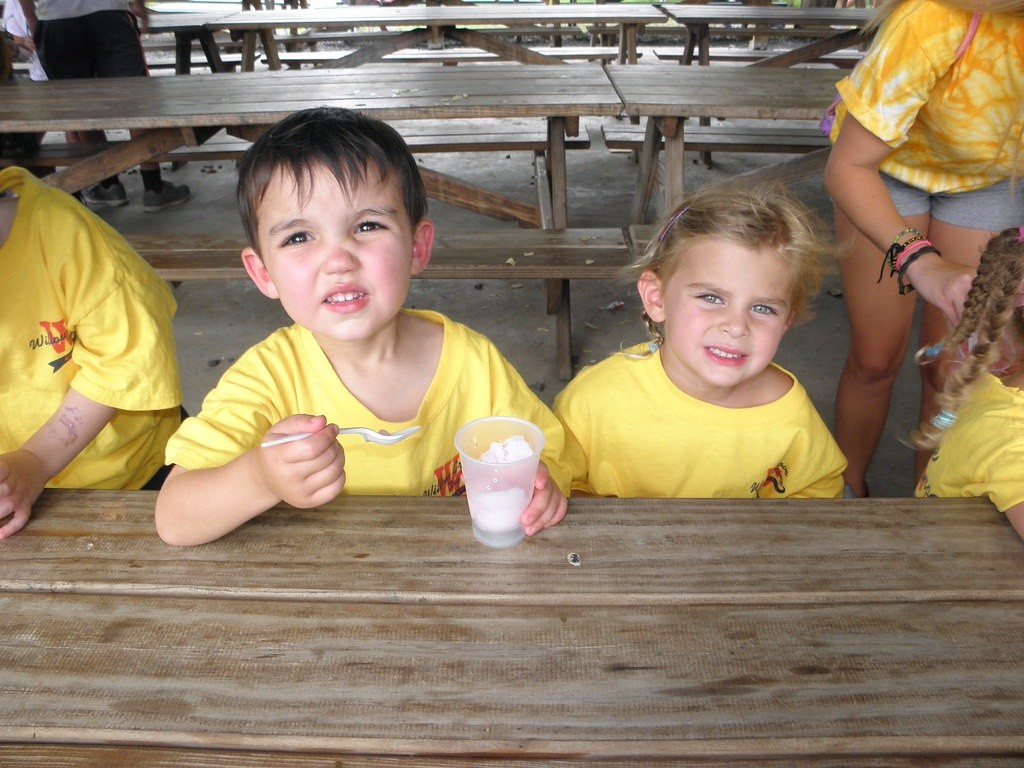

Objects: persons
[19,0,193,215]
[821,0,1023,499]
[907,226,1024,540]
[1,0,81,146]
[0,165,192,540]
[155,104,576,547]
[550,175,848,499]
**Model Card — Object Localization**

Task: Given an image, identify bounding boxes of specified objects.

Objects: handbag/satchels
[820,87,842,136]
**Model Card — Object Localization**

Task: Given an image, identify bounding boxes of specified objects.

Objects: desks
[604,64,855,222]
[0,64,624,382]
[0,487,1024,768]
[660,4,875,164]
[206,4,665,163]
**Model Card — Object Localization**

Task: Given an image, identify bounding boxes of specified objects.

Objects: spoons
[260,426,419,449]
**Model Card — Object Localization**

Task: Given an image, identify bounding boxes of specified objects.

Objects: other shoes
[143,180,191,212]
[81,182,129,207]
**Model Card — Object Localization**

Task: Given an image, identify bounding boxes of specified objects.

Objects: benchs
[653,48,863,62]
[273,26,582,45]
[33,123,590,169]
[127,226,635,281]
[601,123,831,152]
[261,47,642,62]
[587,26,848,36]
[147,53,262,69]
[141,39,259,51]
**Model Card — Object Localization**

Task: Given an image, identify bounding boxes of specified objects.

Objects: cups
[454,418,545,548]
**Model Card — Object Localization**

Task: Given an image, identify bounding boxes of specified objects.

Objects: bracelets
[876,227,942,296]
